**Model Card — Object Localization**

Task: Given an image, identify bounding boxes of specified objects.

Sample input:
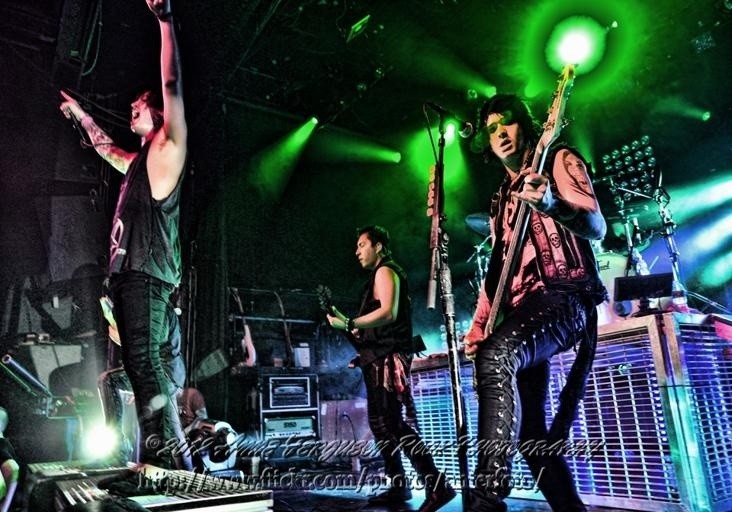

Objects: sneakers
[369,486,412,503]
[420,485,457,511]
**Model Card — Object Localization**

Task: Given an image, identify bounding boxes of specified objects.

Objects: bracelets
[155,11,173,21]
[343,317,352,333]
[77,109,94,125]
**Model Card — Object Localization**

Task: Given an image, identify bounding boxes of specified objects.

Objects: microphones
[426,100,473,139]
[65,106,86,142]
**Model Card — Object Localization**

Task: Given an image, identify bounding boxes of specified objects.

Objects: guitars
[319,288,361,368]
[484,63,576,341]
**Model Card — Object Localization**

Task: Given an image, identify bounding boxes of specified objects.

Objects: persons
[458,92,608,512]
[0,406,22,512]
[170,385,215,469]
[326,225,456,512]
[58,0,192,472]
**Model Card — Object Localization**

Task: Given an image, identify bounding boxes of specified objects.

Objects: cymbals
[466,212,492,236]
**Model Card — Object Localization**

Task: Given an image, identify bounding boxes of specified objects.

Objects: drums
[594,252,651,315]
[602,205,653,256]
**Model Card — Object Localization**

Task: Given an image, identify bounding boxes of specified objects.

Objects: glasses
[488,116,514,134]
